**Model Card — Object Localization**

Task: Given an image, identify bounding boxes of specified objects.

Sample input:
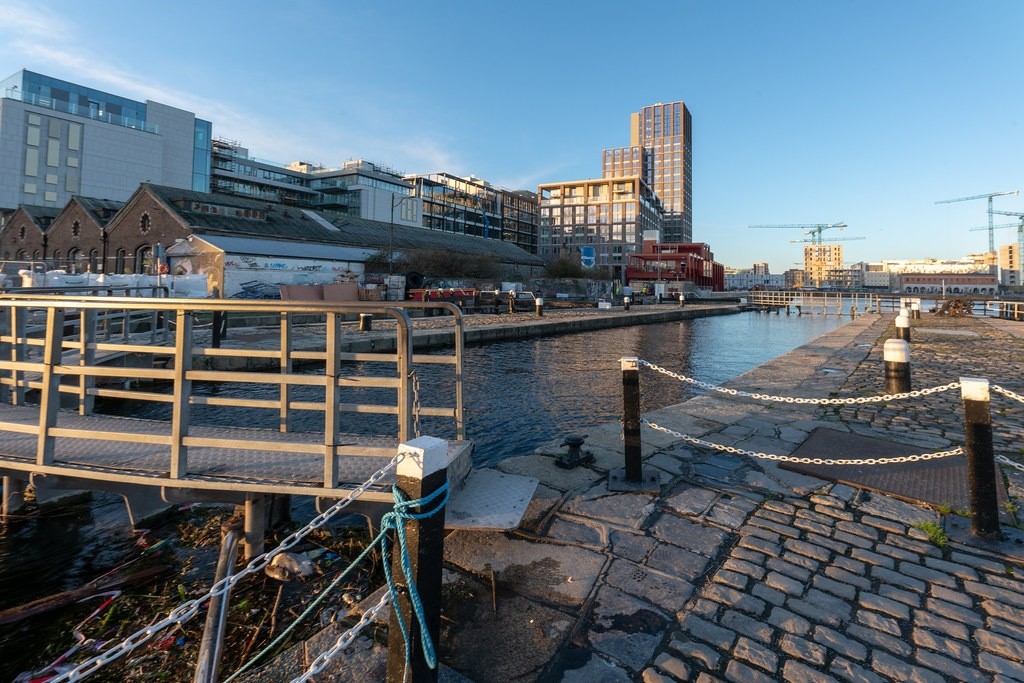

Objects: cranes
[747,220,866,246]
[935,190,1024,251]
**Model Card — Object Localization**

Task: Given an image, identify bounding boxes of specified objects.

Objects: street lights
[388,192,416,274]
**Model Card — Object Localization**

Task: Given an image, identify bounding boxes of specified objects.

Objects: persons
[642,285,651,296]
[616,285,620,294]
